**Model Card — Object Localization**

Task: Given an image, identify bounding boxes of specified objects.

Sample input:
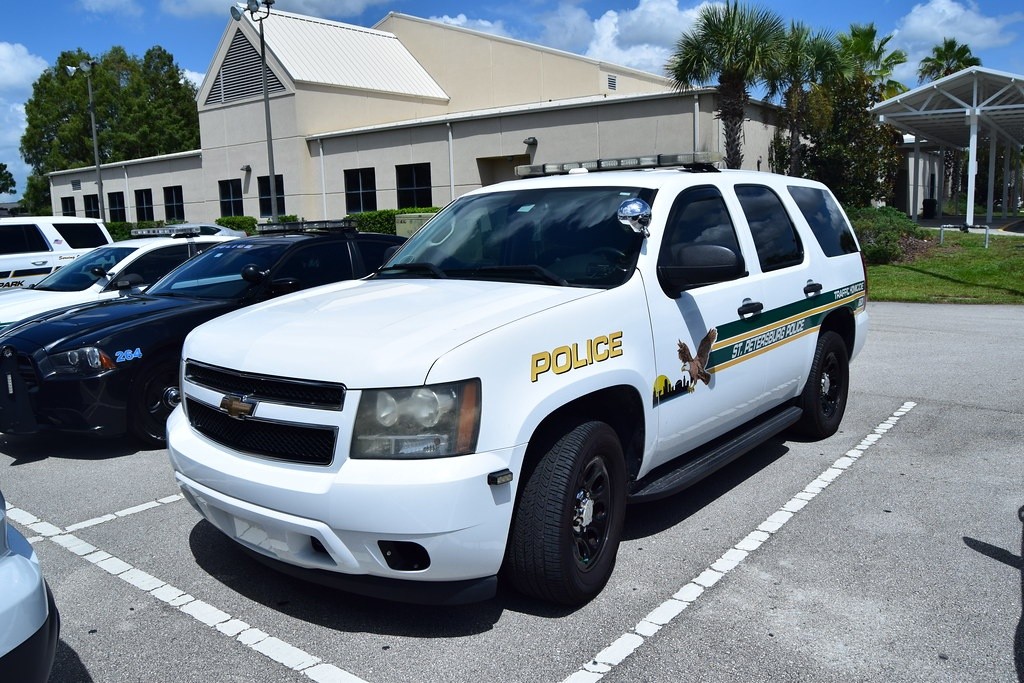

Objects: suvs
[165,163,869,617]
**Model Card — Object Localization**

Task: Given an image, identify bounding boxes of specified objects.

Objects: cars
[0,491,62,683]
[0,215,411,452]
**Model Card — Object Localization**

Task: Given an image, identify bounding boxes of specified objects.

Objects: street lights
[64,57,106,218]
[230,0,277,222]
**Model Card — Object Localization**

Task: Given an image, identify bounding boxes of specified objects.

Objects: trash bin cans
[923,198,936,219]
